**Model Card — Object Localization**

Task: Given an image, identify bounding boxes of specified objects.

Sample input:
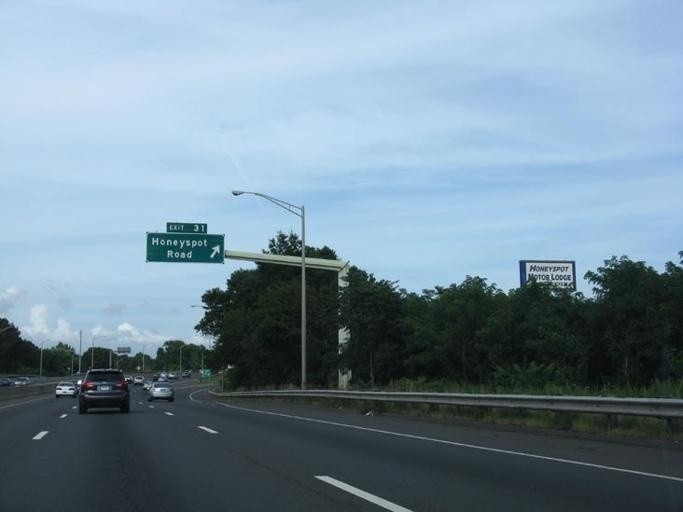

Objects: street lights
[142,342,154,370]
[179,341,189,375]
[188,304,225,392]
[229,189,308,391]
[90,335,107,369]
[39,338,51,378]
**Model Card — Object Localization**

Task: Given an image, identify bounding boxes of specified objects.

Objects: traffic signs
[142,231,227,265]
[163,222,209,235]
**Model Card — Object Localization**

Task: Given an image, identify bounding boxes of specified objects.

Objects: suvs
[76,366,130,414]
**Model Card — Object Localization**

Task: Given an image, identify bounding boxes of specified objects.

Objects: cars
[147,379,174,402]
[0,376,13,387]
[51,368,192,398]
[13,376,32,386]
